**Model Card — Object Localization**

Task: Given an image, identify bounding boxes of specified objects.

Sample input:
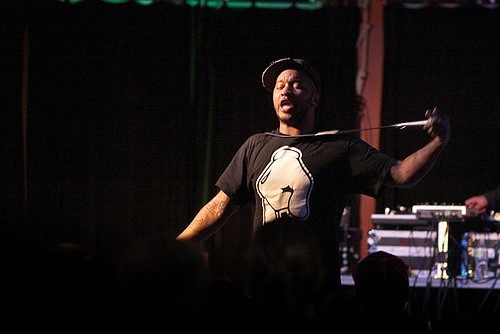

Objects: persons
[176,58,450,285]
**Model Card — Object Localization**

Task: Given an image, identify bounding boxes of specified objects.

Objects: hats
[262,58,321,91]
